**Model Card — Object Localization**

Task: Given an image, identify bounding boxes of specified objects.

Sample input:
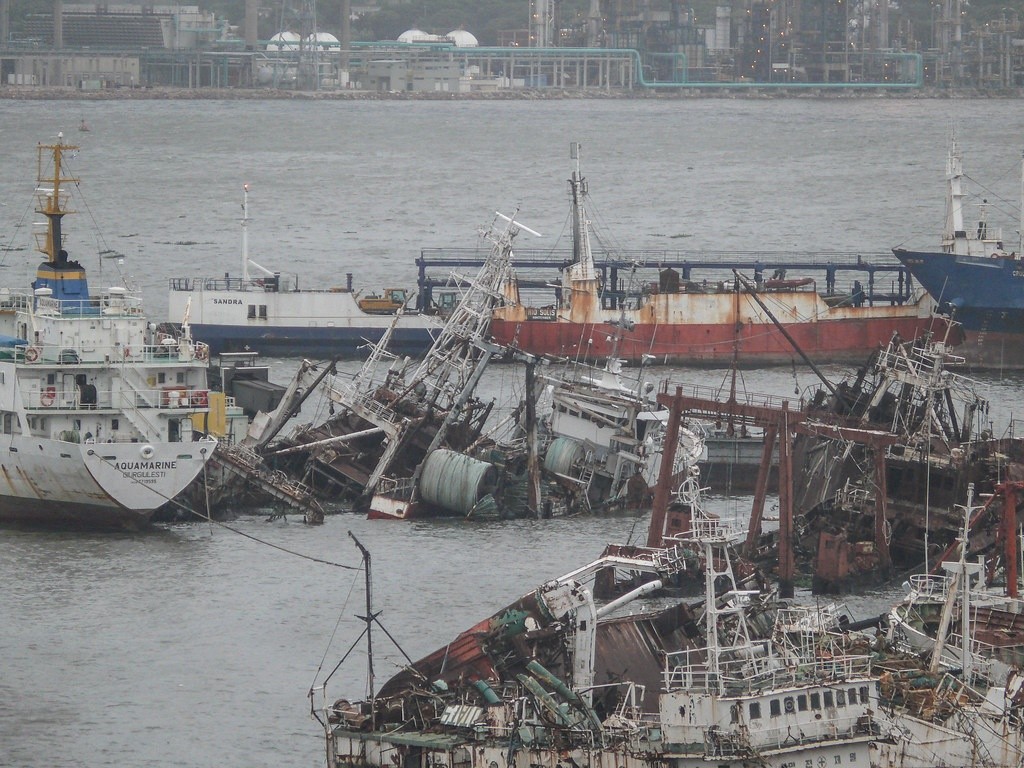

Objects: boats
[222,201,1021,766]
[891,127,1024,343]
[0,133,216,530]
[489,144,958,368]
[164,182,449,357]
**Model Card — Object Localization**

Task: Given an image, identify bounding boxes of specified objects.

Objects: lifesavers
[193,391,206,404]
[25,349,38,361]
[125,348,130,357]
[41,394,55,407]
[197,347,209,359]
